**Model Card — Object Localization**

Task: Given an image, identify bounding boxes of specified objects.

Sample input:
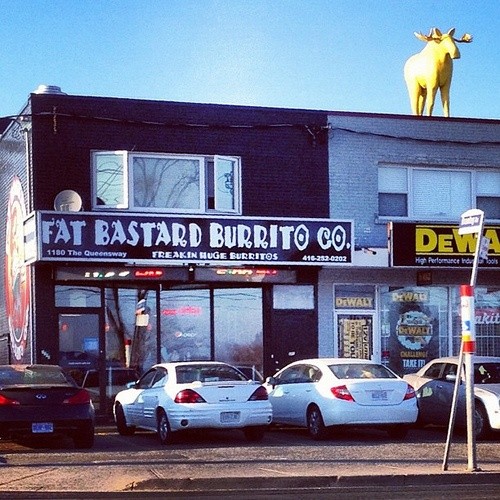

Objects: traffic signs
[134,299,145,313]
[456,208,490,261]
[135,314,149,327]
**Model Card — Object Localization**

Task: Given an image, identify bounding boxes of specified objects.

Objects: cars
[0,364,96,451]
[259,358,418,440]
[113,359,273,443]
[403,356,500,441]
